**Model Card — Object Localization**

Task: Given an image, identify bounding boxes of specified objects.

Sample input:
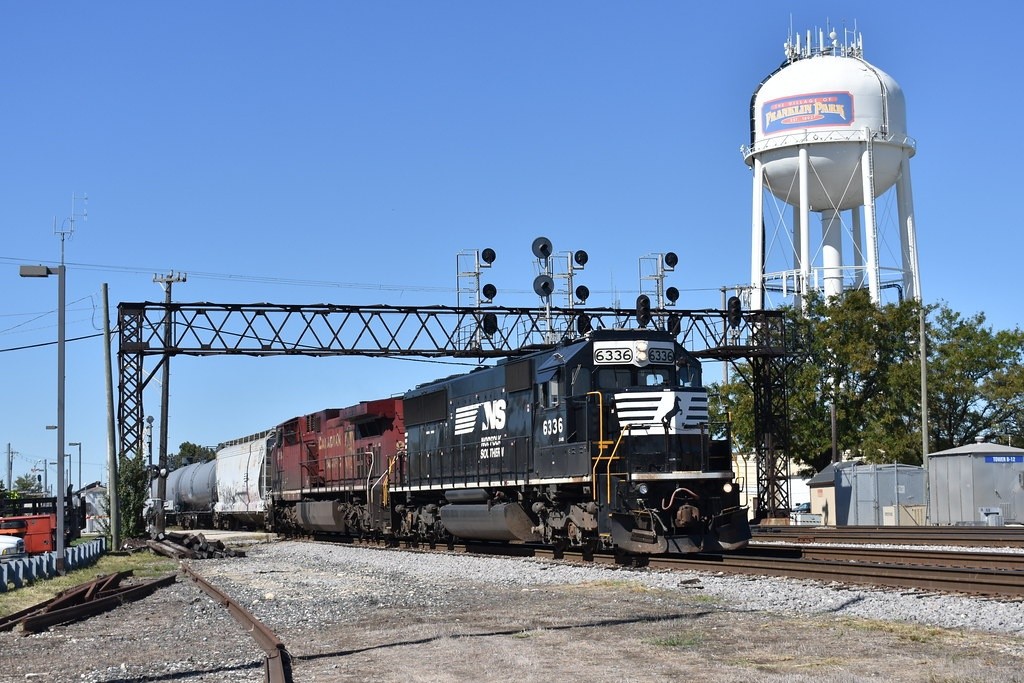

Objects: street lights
[69,442,82,491]
[63,453,72,486]
[19,263,66,576]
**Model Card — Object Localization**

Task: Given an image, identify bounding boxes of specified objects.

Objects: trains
[146,325,755,559]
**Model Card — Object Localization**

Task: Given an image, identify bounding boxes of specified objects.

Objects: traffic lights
[534,275,555,298]
[727,295,743,328]
[636,295,652,325]
[532,236,553,260]
